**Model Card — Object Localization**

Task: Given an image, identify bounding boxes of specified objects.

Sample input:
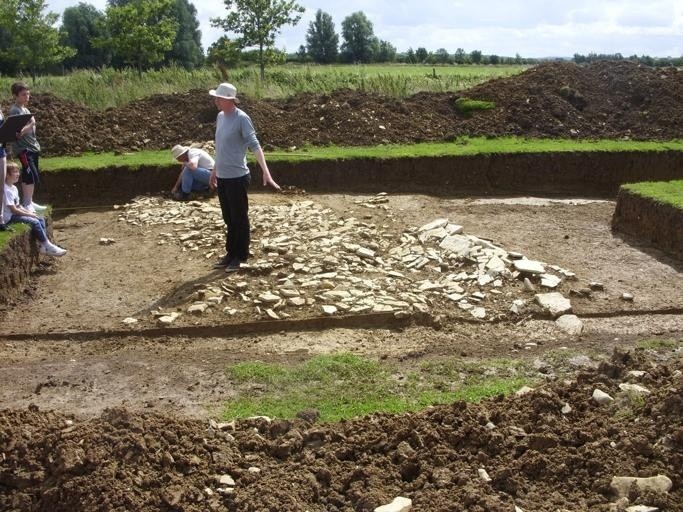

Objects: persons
[7,80,48,214]
[169,144,218,201]
[0,161,66,257]
[207,82,282,272]
[0,109,33,233]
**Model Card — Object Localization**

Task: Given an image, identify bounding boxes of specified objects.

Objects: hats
[208,82,241,104]
[170,145,190,160]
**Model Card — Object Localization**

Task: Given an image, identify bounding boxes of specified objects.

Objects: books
[0,112,35,140]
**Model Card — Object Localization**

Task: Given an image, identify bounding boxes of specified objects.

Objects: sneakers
[23,201,47,213]
[173,191,195,200]
[203,183,218,197]
[39,243,67,256]
[0,223,15,231]
[225,257,247,272]
[213,254,234,267]
